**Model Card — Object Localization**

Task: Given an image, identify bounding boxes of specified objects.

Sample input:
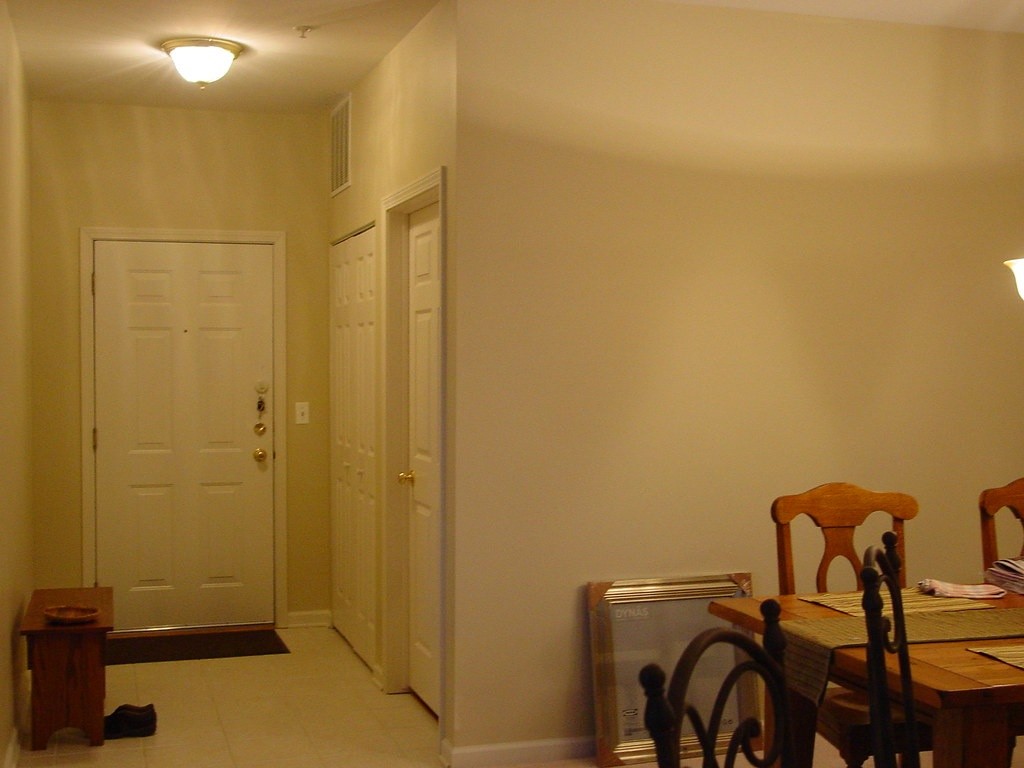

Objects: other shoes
[102,703,157,739]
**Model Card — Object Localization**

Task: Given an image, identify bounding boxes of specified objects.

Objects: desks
[709,583,1024,768]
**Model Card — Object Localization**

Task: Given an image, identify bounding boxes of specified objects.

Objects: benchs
[20,586,113,751]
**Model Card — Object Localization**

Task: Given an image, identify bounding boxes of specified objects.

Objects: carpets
[106,629,292,666]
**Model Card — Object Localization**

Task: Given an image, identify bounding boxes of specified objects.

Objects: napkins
[917,578,1008,598]
[984,559,1024,595]
[797,587,996,620]
[968,645,1024,671]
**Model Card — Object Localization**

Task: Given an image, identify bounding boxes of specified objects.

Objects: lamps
[160,38,244,90]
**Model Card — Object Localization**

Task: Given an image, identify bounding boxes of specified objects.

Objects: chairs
[979,477,1024,569]
[772,482,934,768]
[639,598,796,768]
[861,531,921,768]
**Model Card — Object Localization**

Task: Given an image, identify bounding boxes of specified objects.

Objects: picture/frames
[588,572,764,767]
